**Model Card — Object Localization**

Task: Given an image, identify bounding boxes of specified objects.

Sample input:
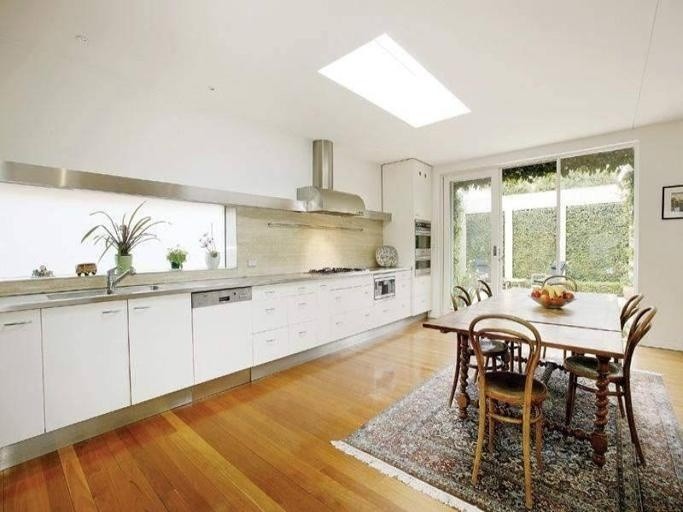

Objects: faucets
[107,265,136,294]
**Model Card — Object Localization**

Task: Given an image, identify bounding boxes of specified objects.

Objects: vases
[205,251,221,269]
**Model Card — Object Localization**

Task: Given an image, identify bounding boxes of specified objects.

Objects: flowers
[198,232,217,257]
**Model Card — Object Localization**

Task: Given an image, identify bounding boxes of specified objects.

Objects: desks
[423,285,650,470]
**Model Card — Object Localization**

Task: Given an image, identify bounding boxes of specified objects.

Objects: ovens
[414,218,432,277]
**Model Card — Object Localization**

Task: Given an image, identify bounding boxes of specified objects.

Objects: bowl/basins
[528,294,578,309]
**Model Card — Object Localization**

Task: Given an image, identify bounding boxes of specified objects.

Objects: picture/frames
[661,184,683,219]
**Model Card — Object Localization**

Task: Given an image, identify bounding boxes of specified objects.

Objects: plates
[375,245,399,267]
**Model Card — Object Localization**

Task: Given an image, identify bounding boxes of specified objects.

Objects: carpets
[329,336,683,512]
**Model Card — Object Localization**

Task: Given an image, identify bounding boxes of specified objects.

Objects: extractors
[295,139,365,216]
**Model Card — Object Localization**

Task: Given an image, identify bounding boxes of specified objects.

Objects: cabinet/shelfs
[0,271,413,472]
[381,159,432,322]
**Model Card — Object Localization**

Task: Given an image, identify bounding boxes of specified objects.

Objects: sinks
[47,284,159,301]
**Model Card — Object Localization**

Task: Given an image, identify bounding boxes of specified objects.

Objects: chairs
[537,276,577,364]
[444,283,520,411]
[569,291,646,420]
[464,313,550,508]
[472,281,524,388]
[563,306,656,469]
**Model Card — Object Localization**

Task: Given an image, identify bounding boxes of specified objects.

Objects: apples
[532,289,573,305]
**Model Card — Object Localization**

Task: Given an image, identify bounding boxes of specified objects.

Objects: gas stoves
[302,266,369,277]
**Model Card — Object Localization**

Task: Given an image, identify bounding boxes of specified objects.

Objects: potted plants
[166,244,190,270]
[80,200,172,274]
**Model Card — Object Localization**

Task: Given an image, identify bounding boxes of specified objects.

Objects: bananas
[542,286,564,299]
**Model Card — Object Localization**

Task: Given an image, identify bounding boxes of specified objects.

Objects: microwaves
[374,275,395,299]
